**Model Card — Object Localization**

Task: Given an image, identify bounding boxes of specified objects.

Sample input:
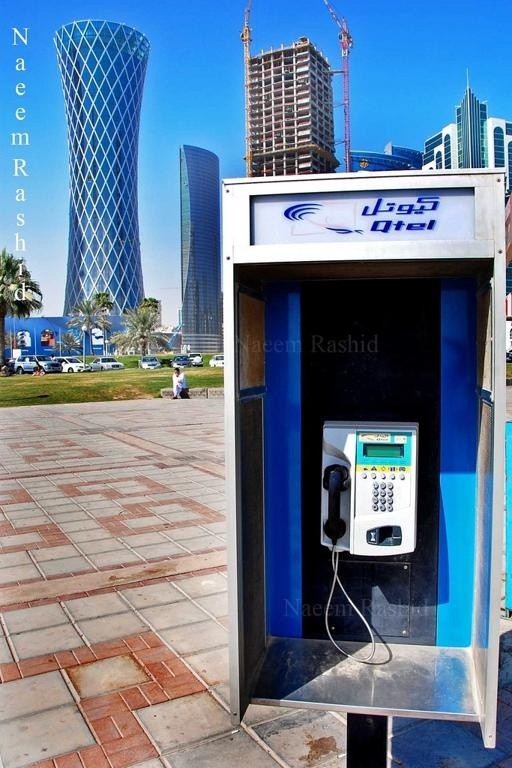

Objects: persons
[172,367,187,399]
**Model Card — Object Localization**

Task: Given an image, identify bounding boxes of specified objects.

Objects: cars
[50,354,91,373]
[169,353,194,368]
[137,355,162,369]
[88,356,125,371]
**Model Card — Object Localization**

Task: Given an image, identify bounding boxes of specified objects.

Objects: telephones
[320,421,419,557]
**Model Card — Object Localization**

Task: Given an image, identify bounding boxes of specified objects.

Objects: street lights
[104,339,109,356]
[81,322,88,369]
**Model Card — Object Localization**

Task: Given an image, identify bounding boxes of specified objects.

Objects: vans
[208,353,224,367]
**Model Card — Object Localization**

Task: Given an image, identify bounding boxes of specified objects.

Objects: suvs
[187,352,204,367]
[12,353,63,374]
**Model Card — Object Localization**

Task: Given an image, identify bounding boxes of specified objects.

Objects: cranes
[238,0,256,179]
[320,0,355,173]
